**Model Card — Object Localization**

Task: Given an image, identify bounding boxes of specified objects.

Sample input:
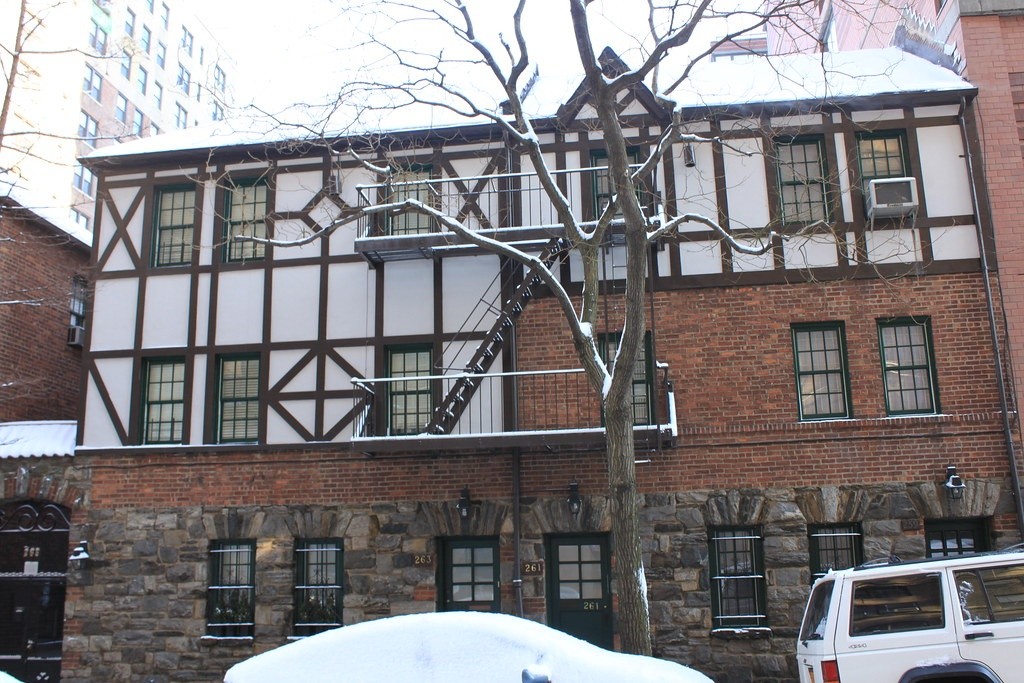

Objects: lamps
[458,490,471,518]
[70,540,89,569]
[567,480,581,514]
[944,466,966,500]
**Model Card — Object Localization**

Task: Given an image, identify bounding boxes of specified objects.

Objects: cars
[219,609,717,683]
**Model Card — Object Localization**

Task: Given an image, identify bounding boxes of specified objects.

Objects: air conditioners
[67,326,85,348]
[866,177,919,219]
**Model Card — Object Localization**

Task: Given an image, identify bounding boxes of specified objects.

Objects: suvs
[793,543,1024,683]
